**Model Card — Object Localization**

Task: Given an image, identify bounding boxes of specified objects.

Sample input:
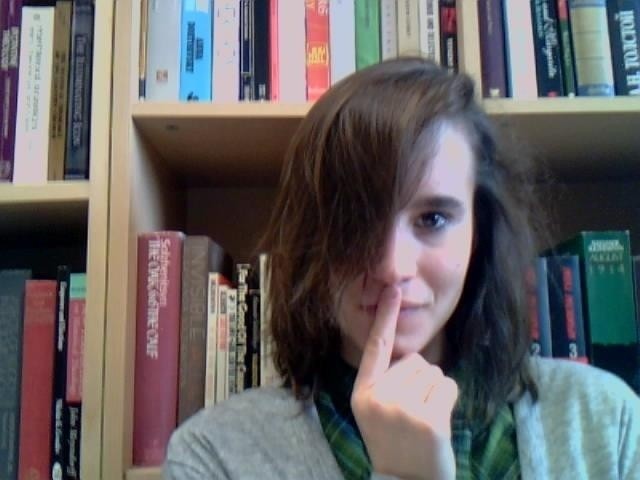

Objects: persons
[158,55,640,480]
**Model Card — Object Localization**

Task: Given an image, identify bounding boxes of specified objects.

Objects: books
[519,228,640,397]
[292,0,640,101]
[0,1,291,480]
